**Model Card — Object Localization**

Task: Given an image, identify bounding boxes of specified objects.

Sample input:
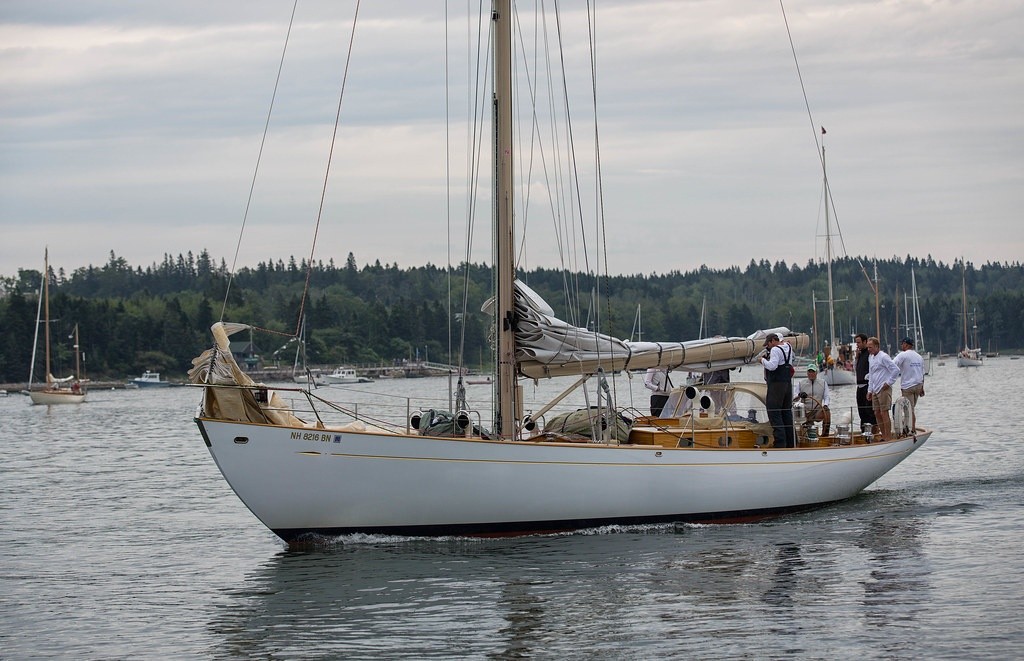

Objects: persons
[855,333,878,436]
[795,364,831,437]
[756,333,798,448]
[645,368,672,417]
[866,337,900,442]
[703,335,736,417]
[892,337,925,435]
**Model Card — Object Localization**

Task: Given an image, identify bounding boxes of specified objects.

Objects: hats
[899,337,913,346]
[807,364,817,371]
[763,334,778,347]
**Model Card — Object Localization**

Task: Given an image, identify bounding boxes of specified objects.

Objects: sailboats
[956,264,984,368]
[570,260,931,380]
[292,314,318,384]
[814,143,856,385]
[20,246,85,405]
[191,0,934,548]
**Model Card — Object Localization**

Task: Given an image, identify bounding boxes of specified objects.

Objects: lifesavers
[894,397,913,436]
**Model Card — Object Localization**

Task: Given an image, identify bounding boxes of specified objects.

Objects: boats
[465,380,492,385]
[321,366,374,384]
[129,371,171,389]
[1011,356,1020,360]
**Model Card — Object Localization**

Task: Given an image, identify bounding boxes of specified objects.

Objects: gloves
[798,392,808,398]
